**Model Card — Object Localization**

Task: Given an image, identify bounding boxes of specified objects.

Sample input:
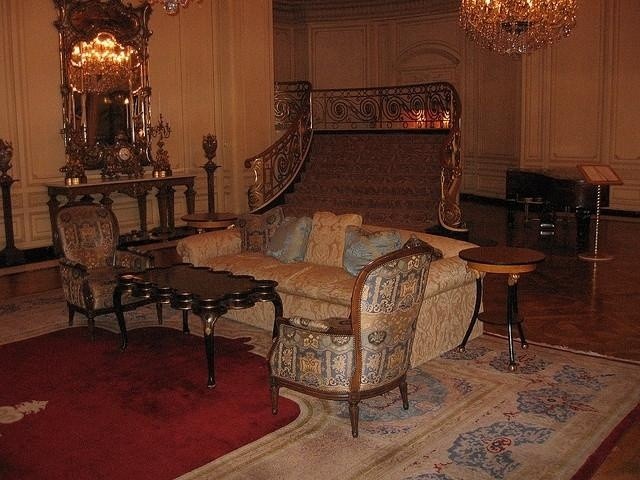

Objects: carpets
[0,283,639,480]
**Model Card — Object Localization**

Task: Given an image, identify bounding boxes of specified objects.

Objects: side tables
[456,240,547,371]
[179,210,238,232]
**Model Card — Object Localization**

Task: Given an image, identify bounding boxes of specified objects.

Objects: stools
[505,196,551,232]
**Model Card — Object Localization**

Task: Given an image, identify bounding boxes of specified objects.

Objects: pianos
[505,167,609,252]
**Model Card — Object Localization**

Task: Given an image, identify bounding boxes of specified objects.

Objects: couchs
[267,243,433,438]
[52,203,166,347]
[174,212,485,367]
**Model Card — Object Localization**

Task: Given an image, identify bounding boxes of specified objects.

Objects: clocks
[102,128,147,182]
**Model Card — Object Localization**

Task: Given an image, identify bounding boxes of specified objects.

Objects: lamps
[455,0,580,55]
[68,29,136,90]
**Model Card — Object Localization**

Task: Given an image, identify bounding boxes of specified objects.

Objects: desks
[45,173,197,253]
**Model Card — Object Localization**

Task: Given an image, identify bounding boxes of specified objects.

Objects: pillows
[303,209,363,271]
[238,205,285,255]
[265,213,311,264]
[342,223,402,277]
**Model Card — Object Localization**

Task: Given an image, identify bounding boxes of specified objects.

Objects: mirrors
[55,2,154,151]
